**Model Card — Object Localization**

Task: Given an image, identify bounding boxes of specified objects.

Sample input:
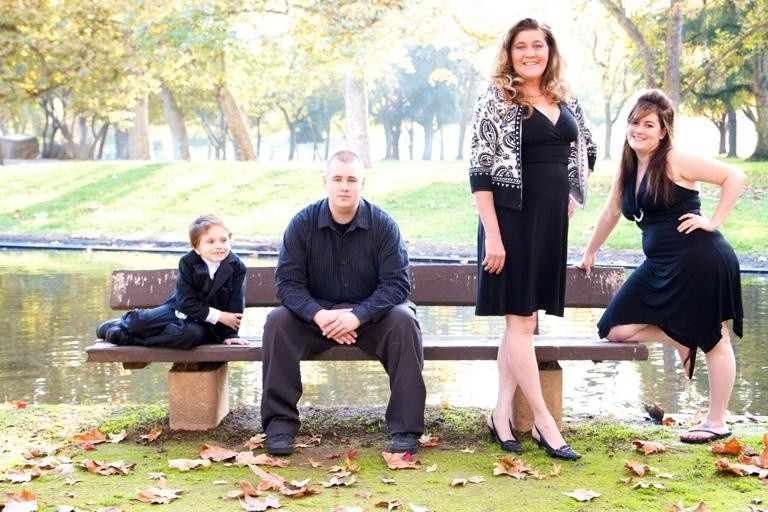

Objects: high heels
[530,422,582,460]
[486,412,525,452]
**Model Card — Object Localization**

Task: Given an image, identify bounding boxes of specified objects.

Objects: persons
[257,149,428,457]
[575,89,747,443]
[467,18,583,461]
[94,212,243,348]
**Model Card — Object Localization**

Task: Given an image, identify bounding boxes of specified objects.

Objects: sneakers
[266,433,295,454]
[390,431,419,453]
[96,315,133,345]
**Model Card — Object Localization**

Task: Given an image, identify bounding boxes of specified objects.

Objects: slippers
[681,343,698,380]
[680,423,732,443]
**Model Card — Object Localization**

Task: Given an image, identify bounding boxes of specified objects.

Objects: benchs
[87,261,649,433]
